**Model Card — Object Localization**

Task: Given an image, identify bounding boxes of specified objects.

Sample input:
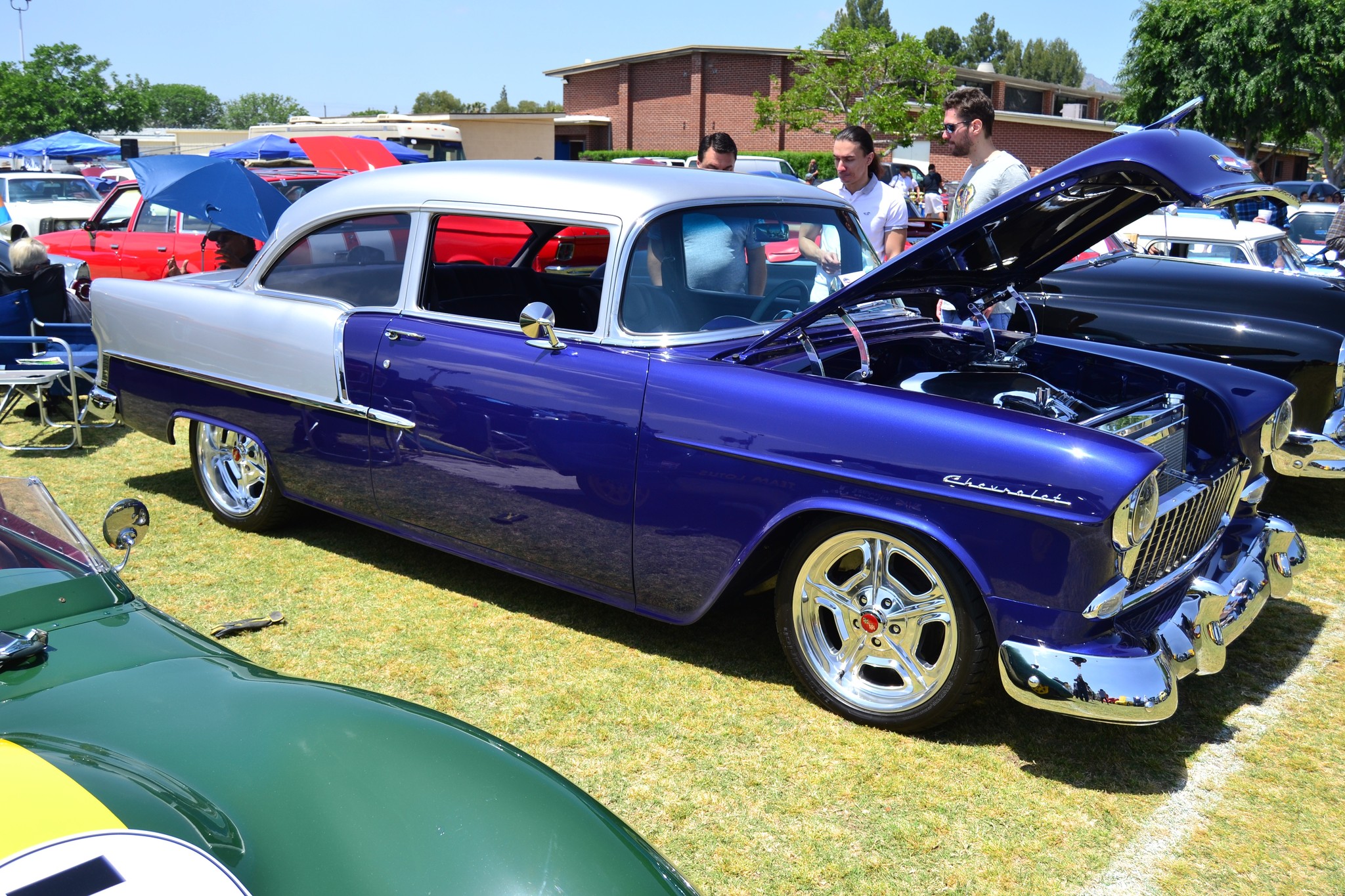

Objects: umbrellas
[126,155,292,243]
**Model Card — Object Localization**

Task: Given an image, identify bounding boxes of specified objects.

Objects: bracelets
[1278,252,1282,255]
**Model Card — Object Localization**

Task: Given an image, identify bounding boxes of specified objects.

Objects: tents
[0,137,45,159]
[13,130,121,171]
[209,133,309,160]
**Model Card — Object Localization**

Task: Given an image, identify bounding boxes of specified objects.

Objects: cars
[0,476,704,896]
[0,114,613,287]
[1055,176,1345,282]
[87,96,1307,736]
[1009,227,1345,487]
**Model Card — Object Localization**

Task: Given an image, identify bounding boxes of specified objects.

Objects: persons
[1325,202,1345,261]
[1323,192,1333,202]
[805,173,815,185]
[1300,191,1308,202]
[1220,160,1290,271]
[167,227,260,276]
[8,238,92,324]
[808,159,819,185]
[923,164,946,221]
[799,125,908,302]
[888,166,913,198]
[1333,192,1343,204]
[1146,202,1178,256]
[647,131,767,296]
[908,171,920,202]
[1310,193,1319,202]
[936,87,1032,330]
[61,155,87,193]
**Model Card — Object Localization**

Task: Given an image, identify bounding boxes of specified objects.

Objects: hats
[805,173,815,180]
[208,227,231,241]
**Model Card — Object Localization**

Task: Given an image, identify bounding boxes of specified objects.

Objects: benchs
[280,258,538,320]
[577,274,680,339]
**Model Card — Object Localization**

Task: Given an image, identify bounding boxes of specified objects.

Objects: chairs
[0,289,96,453]
[0,260,73,336]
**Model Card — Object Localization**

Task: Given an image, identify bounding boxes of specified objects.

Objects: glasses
[215,235,229,248]
[944,119,972,134]
[42,259,51,265]
[1333,196,1339,198]
